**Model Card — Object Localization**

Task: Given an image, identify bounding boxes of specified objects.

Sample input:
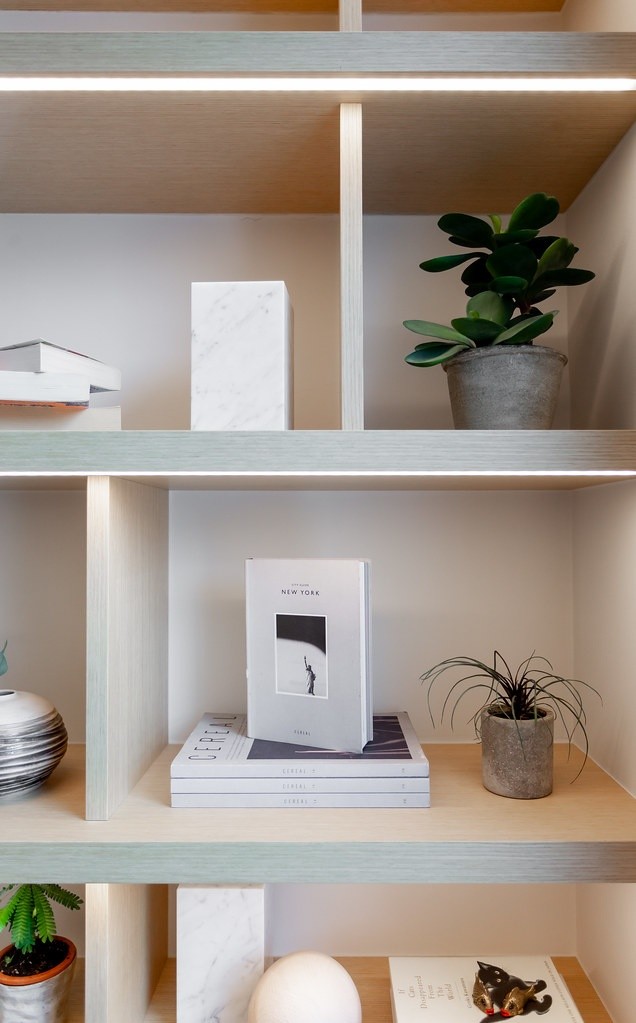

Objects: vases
[0,689,69,802]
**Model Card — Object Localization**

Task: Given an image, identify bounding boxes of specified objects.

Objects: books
[0,337,121,431]
[170,559,431,807]
[388,954,585,1023]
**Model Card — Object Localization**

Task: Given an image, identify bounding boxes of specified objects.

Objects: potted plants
[422,653,603,800]
[0,884,84,1023]
[401,191,597,430]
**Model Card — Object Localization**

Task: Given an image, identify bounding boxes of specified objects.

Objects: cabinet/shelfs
[0,0,636,1023]
